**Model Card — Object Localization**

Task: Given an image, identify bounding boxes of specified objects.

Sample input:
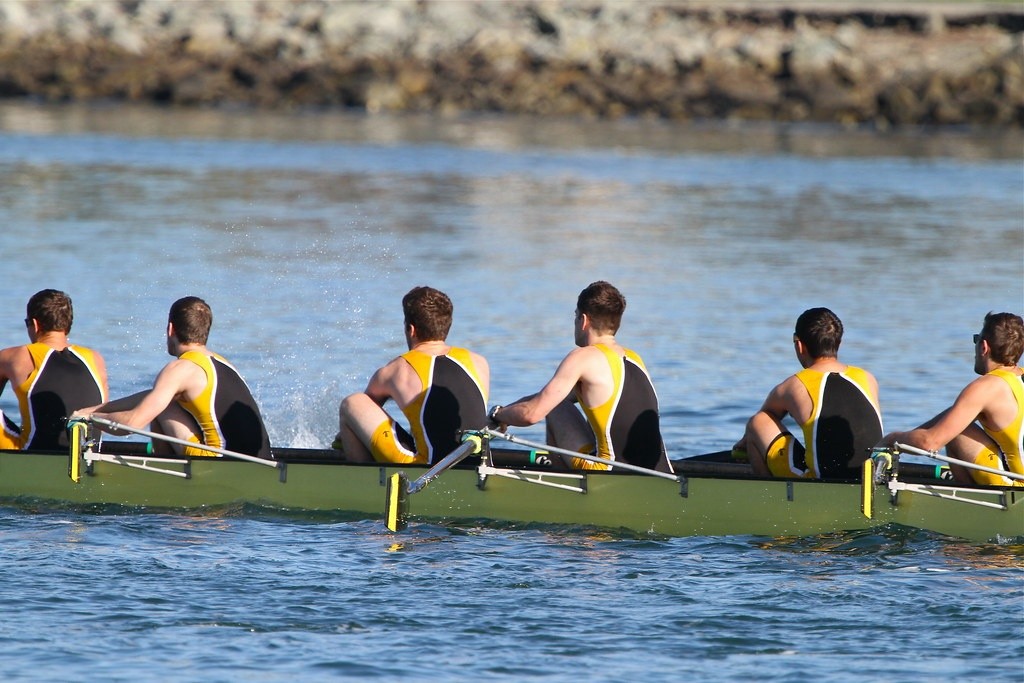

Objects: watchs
[489,405,504,426]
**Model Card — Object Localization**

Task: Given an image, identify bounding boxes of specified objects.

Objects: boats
[0,417,1024,545]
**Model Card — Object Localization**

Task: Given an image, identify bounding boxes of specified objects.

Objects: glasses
[24,318,33,328]
[793,332,801,343]
[973,333,984,344]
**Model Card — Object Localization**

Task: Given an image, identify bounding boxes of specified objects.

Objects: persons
[0,289,110,452]
[733,308,885,475]
[484,280,675,475]
[334,286,490,465]
[71,295,275,460]
[869,310,1024,488]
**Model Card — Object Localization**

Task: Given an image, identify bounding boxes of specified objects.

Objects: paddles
[861,447,892,519]
[384,424,503,533]
[65,418,102,484]
